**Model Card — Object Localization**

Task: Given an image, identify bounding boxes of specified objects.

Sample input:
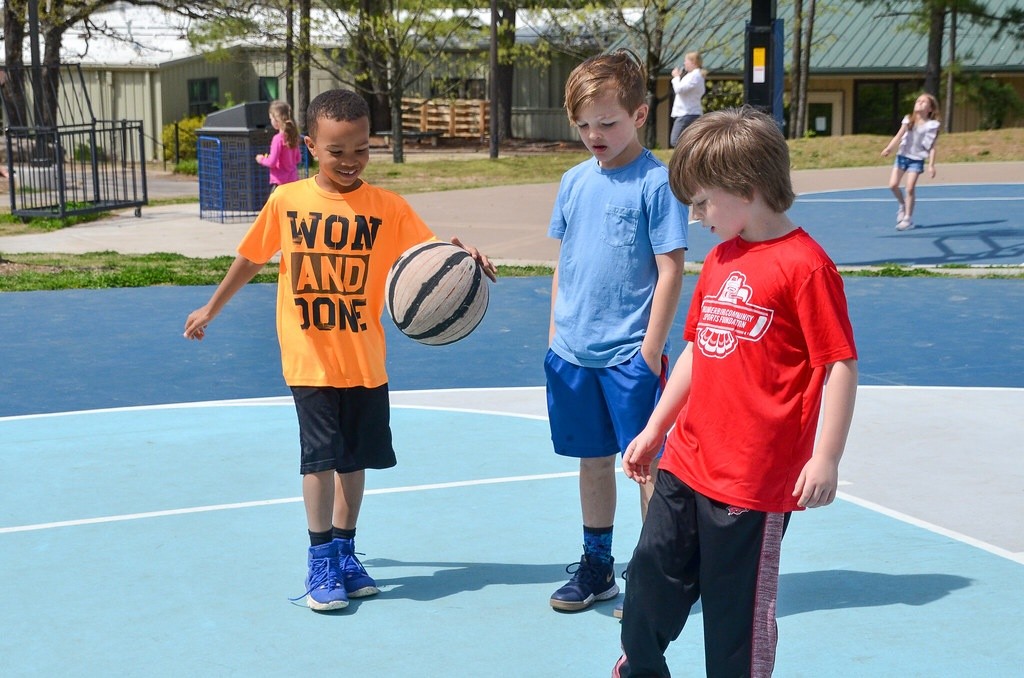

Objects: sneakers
[549,553,621,611]
[895,218,914,231]
[329,537,379,599]
[895,203,904,222]
[303,541,349,611]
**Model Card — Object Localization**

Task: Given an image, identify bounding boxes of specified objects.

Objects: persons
[611,103,859,677]
[183,90,497,612]
[670,52,708,147]
[879,93,941,229]
[539,54,690,613]
[256,99,302,198]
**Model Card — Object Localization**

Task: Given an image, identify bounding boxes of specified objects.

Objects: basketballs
[385,241,490,346]
